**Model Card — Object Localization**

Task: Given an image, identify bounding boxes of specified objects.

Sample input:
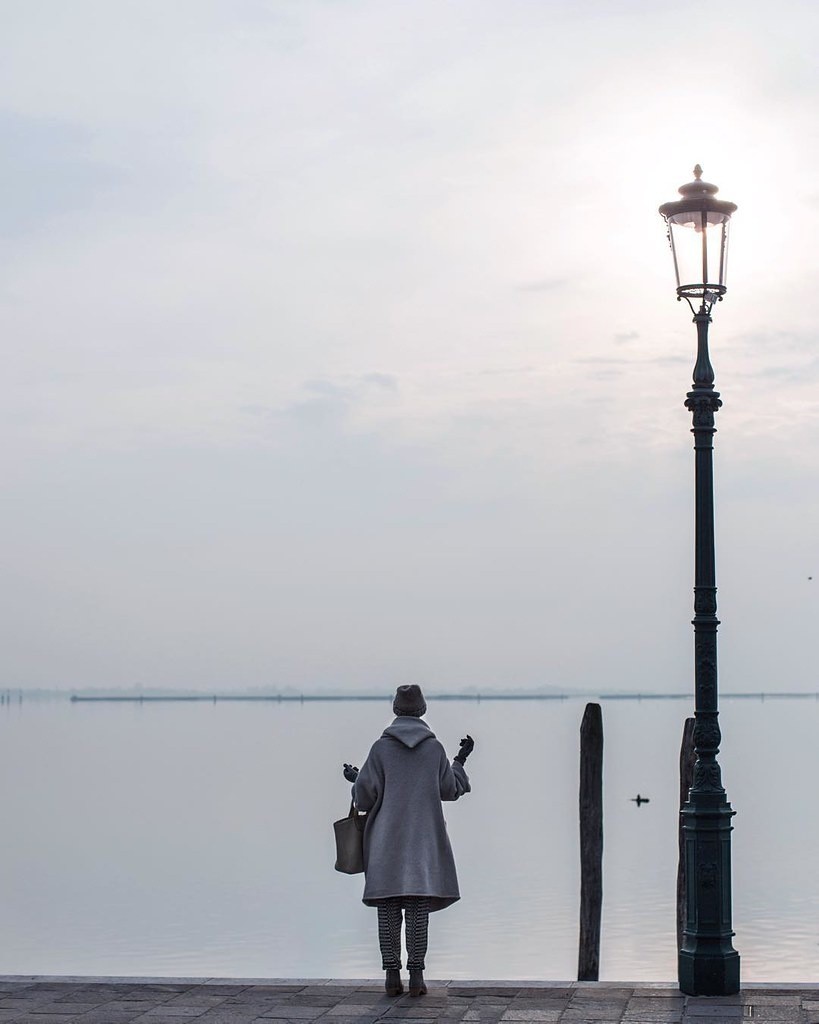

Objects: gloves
[457,735,473,759]
[343,764,358,783]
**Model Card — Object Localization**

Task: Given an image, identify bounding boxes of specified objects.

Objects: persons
[344,684,475,998]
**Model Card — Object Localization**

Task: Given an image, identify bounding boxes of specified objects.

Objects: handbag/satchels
[333,799,368,875]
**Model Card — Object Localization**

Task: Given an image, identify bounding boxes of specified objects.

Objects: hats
[392,685,426,718]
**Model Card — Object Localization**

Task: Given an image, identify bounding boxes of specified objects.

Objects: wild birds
[628,794,650,808]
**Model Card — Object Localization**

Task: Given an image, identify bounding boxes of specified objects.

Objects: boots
[385,970,404,996]
[409,969,427,996]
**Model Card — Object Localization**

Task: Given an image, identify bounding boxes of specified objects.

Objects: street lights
[658,162,744,1000]
